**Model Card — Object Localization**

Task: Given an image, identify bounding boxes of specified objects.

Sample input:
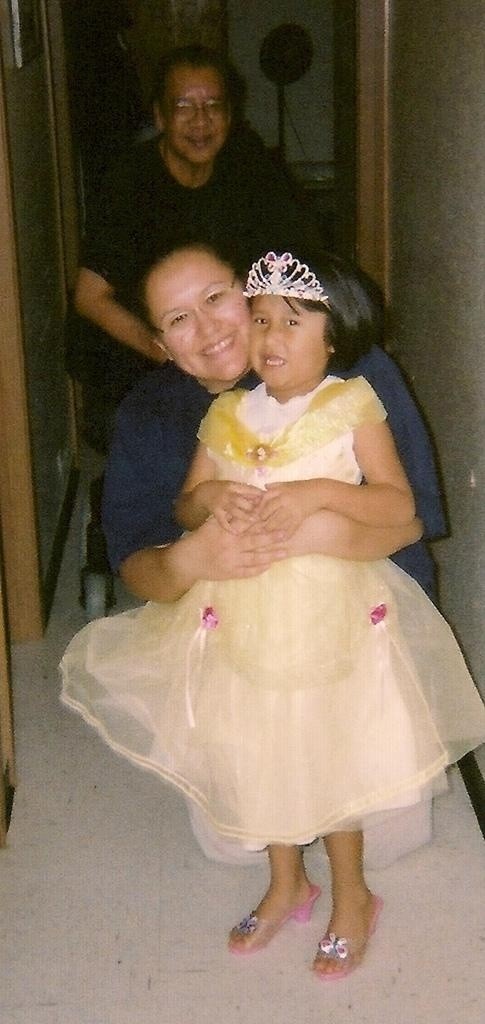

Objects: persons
[54,240,485,981]
[107,233,452,602]
[72,45,299,441]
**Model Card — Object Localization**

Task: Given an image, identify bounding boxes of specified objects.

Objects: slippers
[227,886,324,955]
[313,894,385,981]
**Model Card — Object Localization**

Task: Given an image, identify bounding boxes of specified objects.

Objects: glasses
[170,97,232,127]
[156,277,239,340]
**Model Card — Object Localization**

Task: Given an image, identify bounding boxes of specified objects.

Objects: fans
[258,22,315,194]
